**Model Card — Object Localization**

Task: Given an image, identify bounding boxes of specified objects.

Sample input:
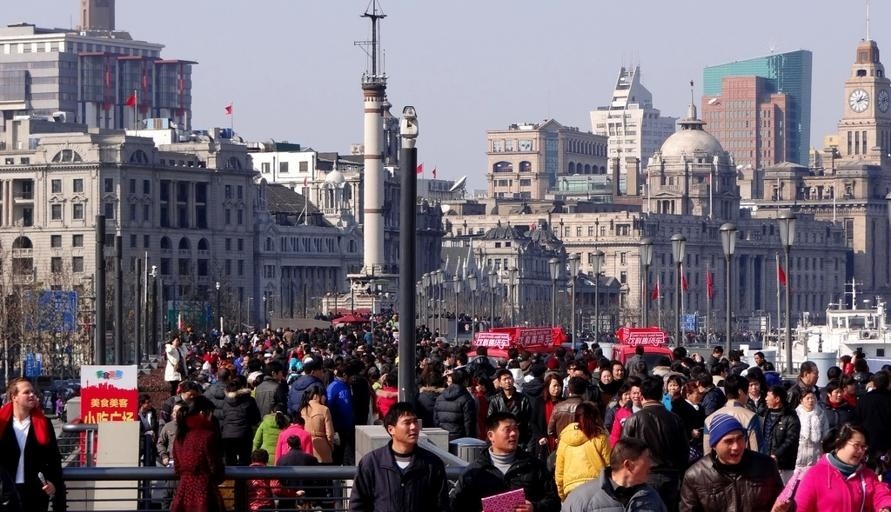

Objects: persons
[416,163,422,174]
[0,306,890,512]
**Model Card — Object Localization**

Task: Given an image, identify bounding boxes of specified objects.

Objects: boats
[740,276,891,389]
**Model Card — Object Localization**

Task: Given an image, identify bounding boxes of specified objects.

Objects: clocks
[881,93,890,114]
[850,90,865,112]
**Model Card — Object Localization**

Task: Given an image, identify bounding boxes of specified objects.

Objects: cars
[0,379,80,413]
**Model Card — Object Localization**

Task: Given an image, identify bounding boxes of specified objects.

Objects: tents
[778,264,786,289]
[706,269,714,300]
[126,95,134,108]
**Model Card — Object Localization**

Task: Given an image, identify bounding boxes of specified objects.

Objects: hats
[708,414,742,448]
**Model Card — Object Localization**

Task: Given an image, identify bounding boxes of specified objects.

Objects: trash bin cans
[457,443,487,464]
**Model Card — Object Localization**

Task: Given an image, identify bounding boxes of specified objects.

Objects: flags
[651,284,656,301]
[224,104,230,115]
[432,169,434,177]
[681,273,689,292]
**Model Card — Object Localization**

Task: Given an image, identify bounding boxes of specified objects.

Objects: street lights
[216,281,222,329]
[417,269,444,338]
[669,234,688,350]
[659,308,821,331]
[778,212,798,377]
[351,281,358,312]
[719,222,740,355]
[567,254,580,349]
[549,258,561,327]
[638,238,654,329]
[468,274,478,338]
[488,270,498,327]
[247,295,254,326]
[453,278,462,346]
[508,267,519,326]
[590,250,605,345]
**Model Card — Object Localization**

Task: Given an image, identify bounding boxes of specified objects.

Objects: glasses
[847,440,867,451]
